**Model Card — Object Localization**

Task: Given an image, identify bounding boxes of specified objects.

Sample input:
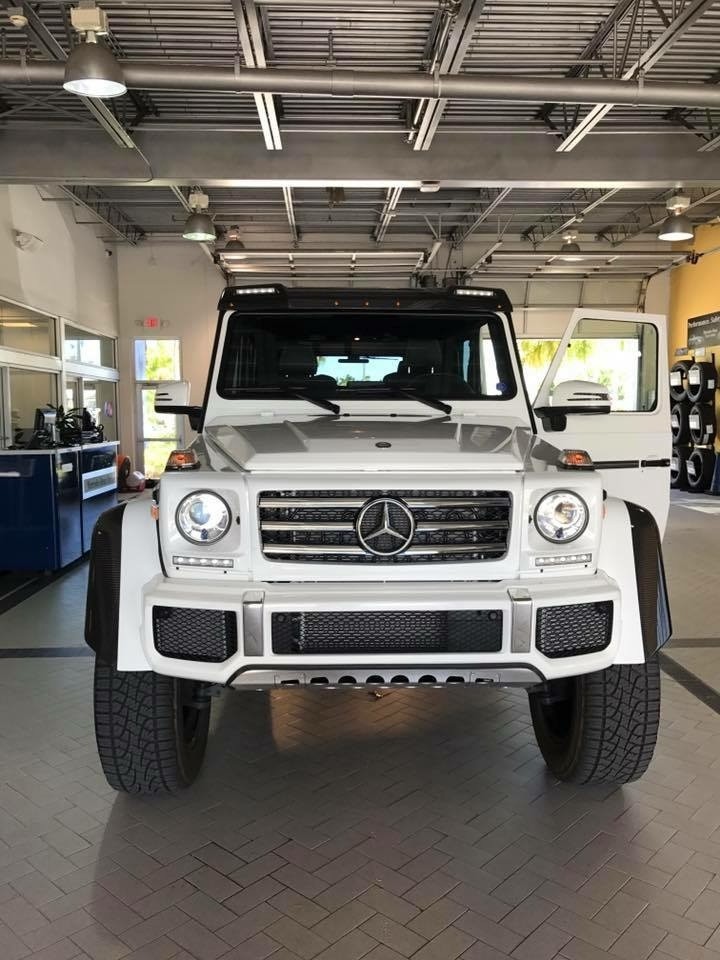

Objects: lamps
[182,185,217,242]
[657,190,695,242]
[63,0,127,98]
[562,230,580,251]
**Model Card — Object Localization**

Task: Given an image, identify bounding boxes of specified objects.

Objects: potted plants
[47,402,105,444]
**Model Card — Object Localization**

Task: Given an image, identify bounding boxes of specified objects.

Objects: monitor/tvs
[33,408,57,443]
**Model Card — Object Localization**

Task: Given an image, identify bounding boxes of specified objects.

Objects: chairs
[384,338,443,383]
[278,349,336,388]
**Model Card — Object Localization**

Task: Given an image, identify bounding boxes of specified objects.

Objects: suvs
[84,269,675,796]
